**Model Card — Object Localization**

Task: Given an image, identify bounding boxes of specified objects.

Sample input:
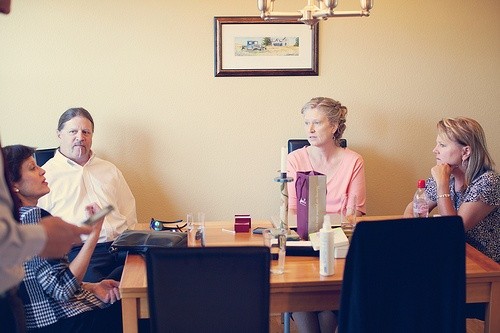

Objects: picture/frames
[214,16,319,76]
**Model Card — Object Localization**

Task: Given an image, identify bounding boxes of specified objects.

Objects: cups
[185,212,205,247]
[263,228,287,274]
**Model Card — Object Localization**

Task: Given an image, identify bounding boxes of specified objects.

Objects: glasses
[149,218,187,231]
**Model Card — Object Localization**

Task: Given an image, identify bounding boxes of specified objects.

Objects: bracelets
[436,194,451,199]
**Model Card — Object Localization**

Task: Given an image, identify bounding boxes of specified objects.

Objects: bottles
[319,214,335,277]
[412,179,428,218]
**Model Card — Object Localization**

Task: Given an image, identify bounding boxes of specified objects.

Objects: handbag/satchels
[295,170,327,241]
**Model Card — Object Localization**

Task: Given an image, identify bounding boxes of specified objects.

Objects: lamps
[257,0,374,29]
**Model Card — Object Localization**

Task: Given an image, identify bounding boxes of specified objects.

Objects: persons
[35,108,139,282]
[286,96,366,333]
[401,117,500,322]
[1,145,150,333]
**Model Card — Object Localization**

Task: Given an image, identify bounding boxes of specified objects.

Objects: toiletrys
[319,214,335,276]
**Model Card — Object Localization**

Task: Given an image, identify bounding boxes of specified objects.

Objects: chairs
[145,246,271,333]
[336,215,466,333]
[281,139,347,333]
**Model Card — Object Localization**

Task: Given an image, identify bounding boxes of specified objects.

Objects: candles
[280,148,287,173]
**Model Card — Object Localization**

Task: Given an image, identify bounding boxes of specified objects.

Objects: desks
[119,214,500,333]
